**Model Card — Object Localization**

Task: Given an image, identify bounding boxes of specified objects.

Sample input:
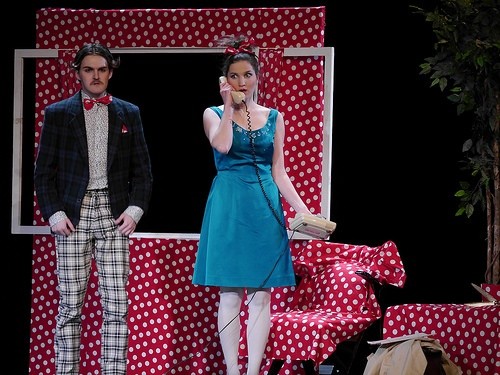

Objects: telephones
[218,76,246,105]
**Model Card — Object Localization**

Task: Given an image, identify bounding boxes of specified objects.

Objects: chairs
[243,260,382,375]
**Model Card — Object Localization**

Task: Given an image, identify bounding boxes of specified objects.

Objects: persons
[33,43,151,375]
[192,54,315,375]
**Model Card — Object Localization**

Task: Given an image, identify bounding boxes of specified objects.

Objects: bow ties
[83,94,112,111]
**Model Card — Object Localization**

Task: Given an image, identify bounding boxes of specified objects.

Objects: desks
[383,303,500,375]
